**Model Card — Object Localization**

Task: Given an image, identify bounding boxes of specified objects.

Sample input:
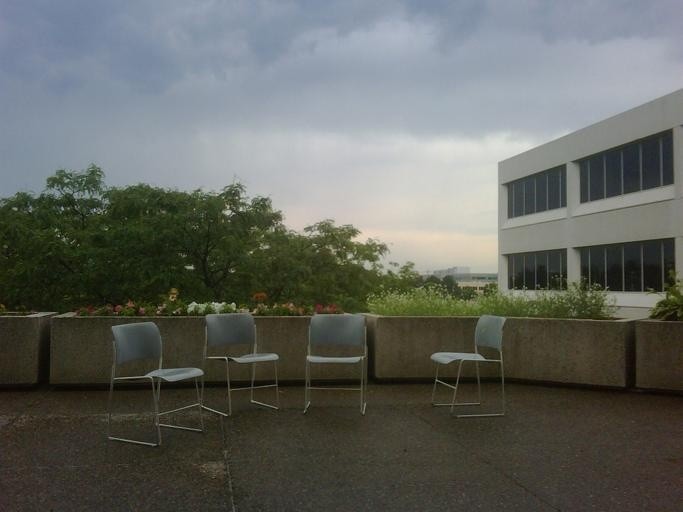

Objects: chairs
[428,313,507,420]
[105,312,369,448]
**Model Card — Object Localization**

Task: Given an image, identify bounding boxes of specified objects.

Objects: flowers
[365,272,622,319]
[76,286,344,314]
